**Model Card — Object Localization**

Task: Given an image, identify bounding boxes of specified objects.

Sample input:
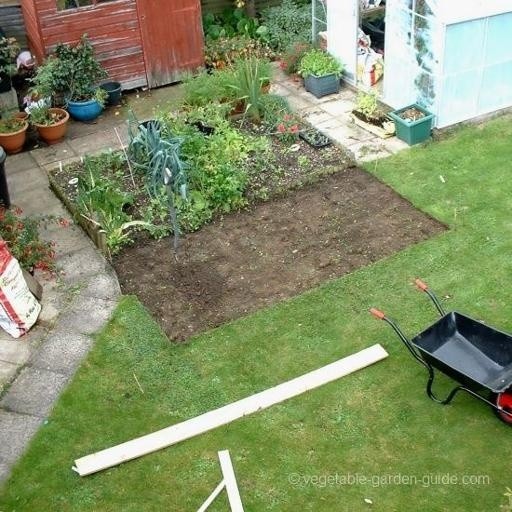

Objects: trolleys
[370,279,511,425]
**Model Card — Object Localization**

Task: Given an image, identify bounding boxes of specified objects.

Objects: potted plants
[351,85,395,139]
[183,57,271,114]
[297,46,343,97]
[0,32,122,155]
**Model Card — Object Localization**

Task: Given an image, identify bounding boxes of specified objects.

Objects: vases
[388,103,435,146]
